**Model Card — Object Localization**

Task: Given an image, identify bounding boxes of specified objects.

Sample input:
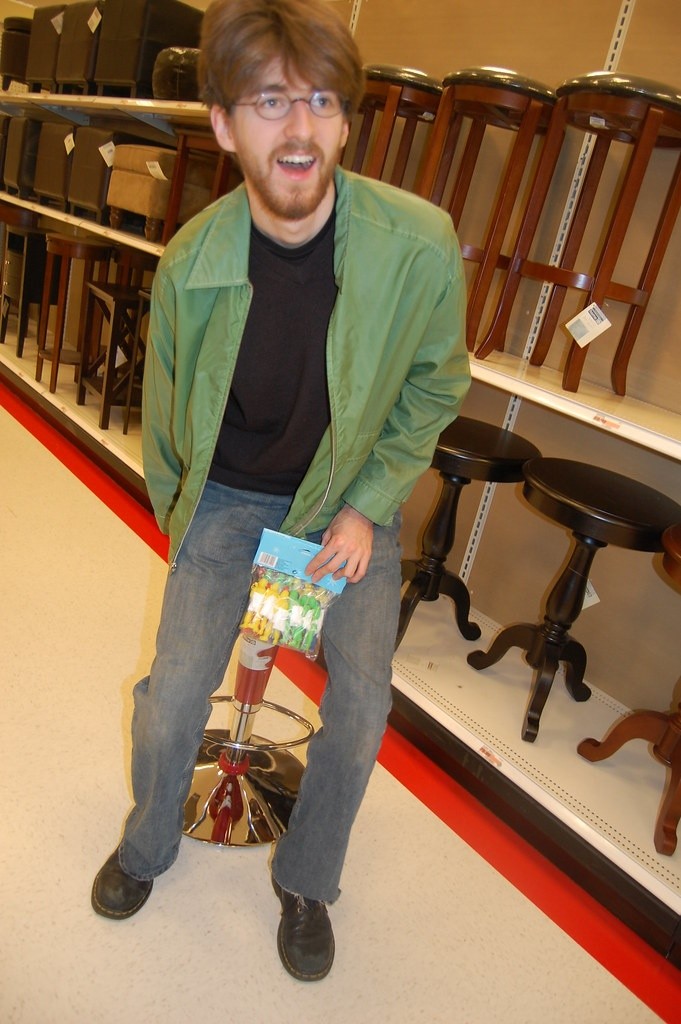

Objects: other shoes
[91,845,153,920]
[272,874,336,983]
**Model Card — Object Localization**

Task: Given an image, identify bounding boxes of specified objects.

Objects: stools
[465,457,681,742]
[0,0,200,100]
[0,103,230,246]
[340,63,443,190]
[577,519,681,856]
[180,634,312,845]
[412,67,557,354]
[476,70,680,392]
[392,415,543,653]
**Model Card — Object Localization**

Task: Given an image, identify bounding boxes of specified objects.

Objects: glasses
[229,90,349,119]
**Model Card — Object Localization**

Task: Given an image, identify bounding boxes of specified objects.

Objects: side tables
[0,199,159,436]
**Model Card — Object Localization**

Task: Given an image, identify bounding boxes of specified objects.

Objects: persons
[92,0,472,981]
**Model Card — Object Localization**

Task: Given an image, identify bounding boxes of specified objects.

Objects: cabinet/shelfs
[0,185,681,926]
